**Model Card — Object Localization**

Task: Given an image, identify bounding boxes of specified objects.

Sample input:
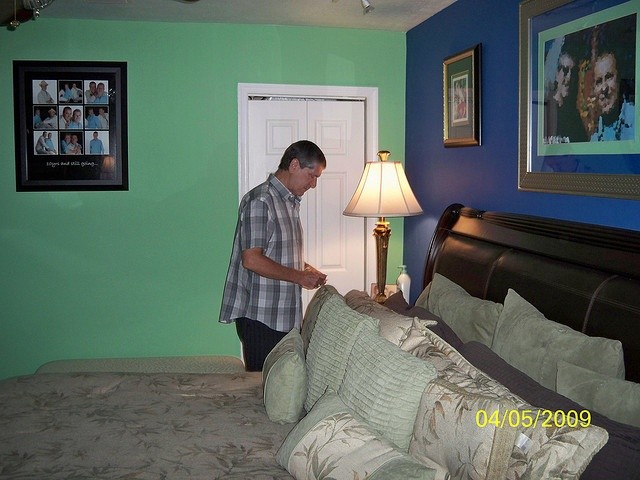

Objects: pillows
[262,326,307,425]
[379,290,464,350]
[557,360,640,427]
[399,315,610,480]
[306,294,379,415]
[344,290,438,348]
[415,271,505,349]
[407,378,518,480]
[274,386,448,480]
[297,285,340,349]
[490,288,626,398]
[337,322,438,454]
[457,341,639,480]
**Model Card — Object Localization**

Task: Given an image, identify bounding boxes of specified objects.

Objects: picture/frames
[517,0,638,199]
[443,45,481,147]
[12,58,128,192]
[370,283,400,302]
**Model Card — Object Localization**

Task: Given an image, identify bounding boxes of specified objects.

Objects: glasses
[558,65,580,74]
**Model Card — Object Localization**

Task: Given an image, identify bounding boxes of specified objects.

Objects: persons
[59,84,72,102]
[35,131,49,154]
[89,132,104,154]
[66,134,82,154]
[34,109,43,129]
[85,81,99,103]
[218,140,327,374]
[44,133,57,154]
[98,109,109,128]
[544,42,588,143]
[87,108,102,128]
[43,108,57,129]
[68,109,82,128]
[71,84,83,102]
[589,42,635,142]
[62,133,82,154]
[59,107,72,128]
[95,83,108,103]
[38,81,55,104]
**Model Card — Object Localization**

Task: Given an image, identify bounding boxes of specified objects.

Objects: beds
[0,202,639,480]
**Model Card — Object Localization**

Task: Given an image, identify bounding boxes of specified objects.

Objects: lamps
[344,151,423,304]
[362,0,374,16]
